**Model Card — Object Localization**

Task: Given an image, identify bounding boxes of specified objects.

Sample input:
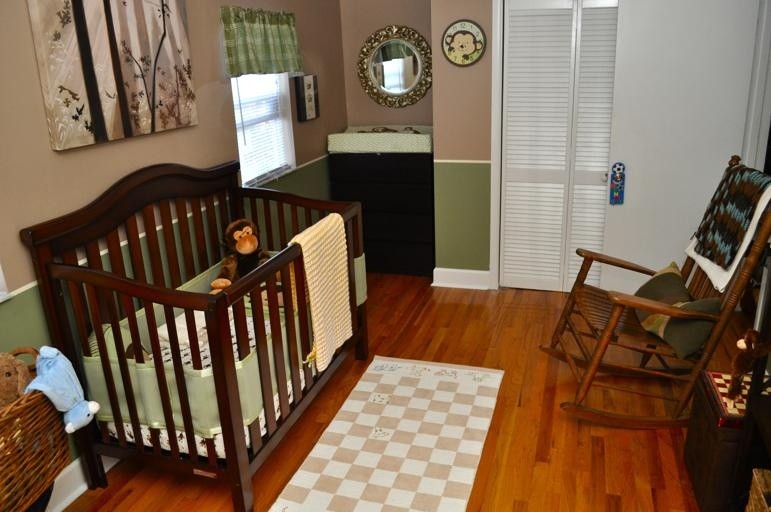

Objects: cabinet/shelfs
[684,368,771,511]
[329,153,435,278]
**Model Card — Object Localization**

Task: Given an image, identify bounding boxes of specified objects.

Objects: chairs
[538,155,771,430]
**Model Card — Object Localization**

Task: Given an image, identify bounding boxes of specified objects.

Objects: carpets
[268,354,507,511]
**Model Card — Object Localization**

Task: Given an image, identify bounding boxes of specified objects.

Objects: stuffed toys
[25,345,100,434]
[209,217,292,290]
[727,328,771,400]
[0,351,27,407]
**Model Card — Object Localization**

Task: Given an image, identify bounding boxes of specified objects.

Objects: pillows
[632,260,723,360]
[157,307,232,344]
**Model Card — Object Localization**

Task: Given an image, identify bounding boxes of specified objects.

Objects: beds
[19,159,371,512]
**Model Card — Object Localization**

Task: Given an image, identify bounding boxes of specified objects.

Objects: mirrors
[358,24,433,110]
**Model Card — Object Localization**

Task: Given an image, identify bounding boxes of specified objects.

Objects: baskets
[1,347,72,512]
